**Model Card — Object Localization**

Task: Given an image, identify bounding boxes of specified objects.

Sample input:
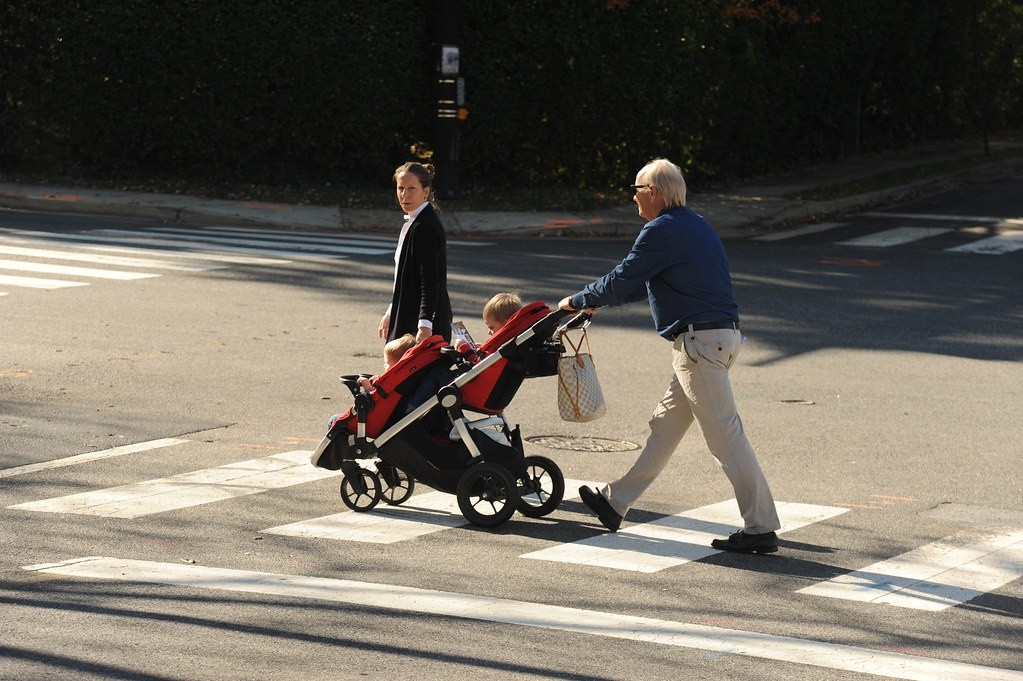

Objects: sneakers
[712,530,779,553]
[579,486,623,533]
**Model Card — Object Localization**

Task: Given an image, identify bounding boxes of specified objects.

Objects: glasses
[631,184,658,195]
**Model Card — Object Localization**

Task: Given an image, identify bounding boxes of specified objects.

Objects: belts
[672,322,740,343]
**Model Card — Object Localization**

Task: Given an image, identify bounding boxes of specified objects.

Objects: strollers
[310,303,596,526]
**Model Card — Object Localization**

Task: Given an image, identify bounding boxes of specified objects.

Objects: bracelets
[568,297,575,310]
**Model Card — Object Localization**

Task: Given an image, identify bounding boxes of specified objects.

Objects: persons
[558,156,782,556]
[455,292,523,353]
[376,161,454,351]
[357,333,417,392]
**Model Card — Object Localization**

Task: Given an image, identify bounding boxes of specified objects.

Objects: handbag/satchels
[557,327,606,422]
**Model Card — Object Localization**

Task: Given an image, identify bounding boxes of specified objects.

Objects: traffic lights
[458,105,469,121]
